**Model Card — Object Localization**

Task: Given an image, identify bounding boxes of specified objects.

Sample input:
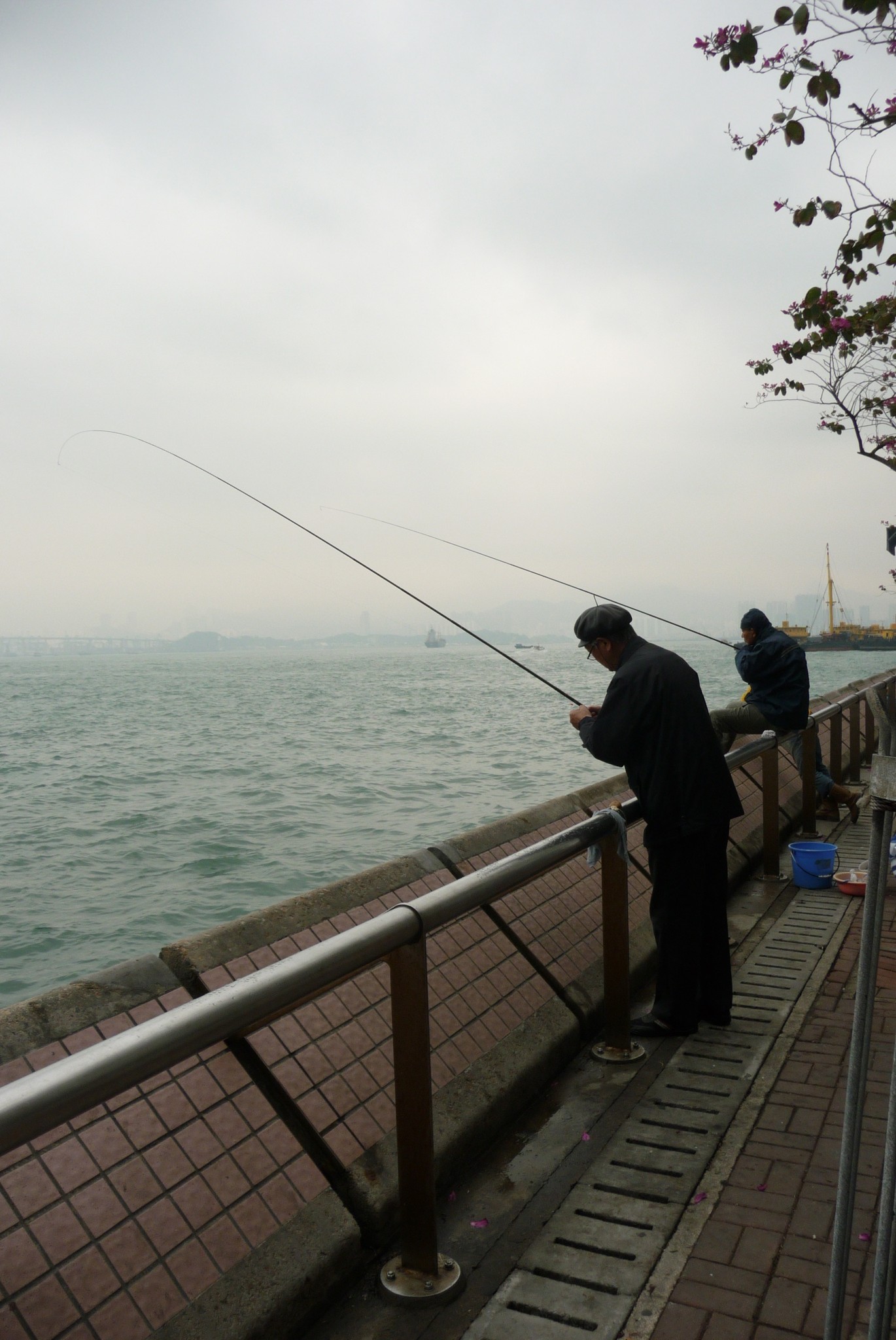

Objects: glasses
[587,642,597,662]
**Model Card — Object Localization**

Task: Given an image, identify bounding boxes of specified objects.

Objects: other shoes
[843,790,865,825]
[814,801,842,823]
[630,1012,732,1037]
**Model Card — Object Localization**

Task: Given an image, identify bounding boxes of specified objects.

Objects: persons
[568,604,737,1043]
[707,608,811,757]
[740,685,863,825]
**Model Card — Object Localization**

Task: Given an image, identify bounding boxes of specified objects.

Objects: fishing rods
[54,426,587,709]
[314,491,742,652]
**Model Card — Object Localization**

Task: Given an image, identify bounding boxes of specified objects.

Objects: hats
[740,608,771,630]
[573,604,632,647]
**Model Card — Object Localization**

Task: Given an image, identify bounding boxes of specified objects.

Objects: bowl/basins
[832,872,868,896]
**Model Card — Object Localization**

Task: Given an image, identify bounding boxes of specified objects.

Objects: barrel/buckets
[789,841,839,890]
